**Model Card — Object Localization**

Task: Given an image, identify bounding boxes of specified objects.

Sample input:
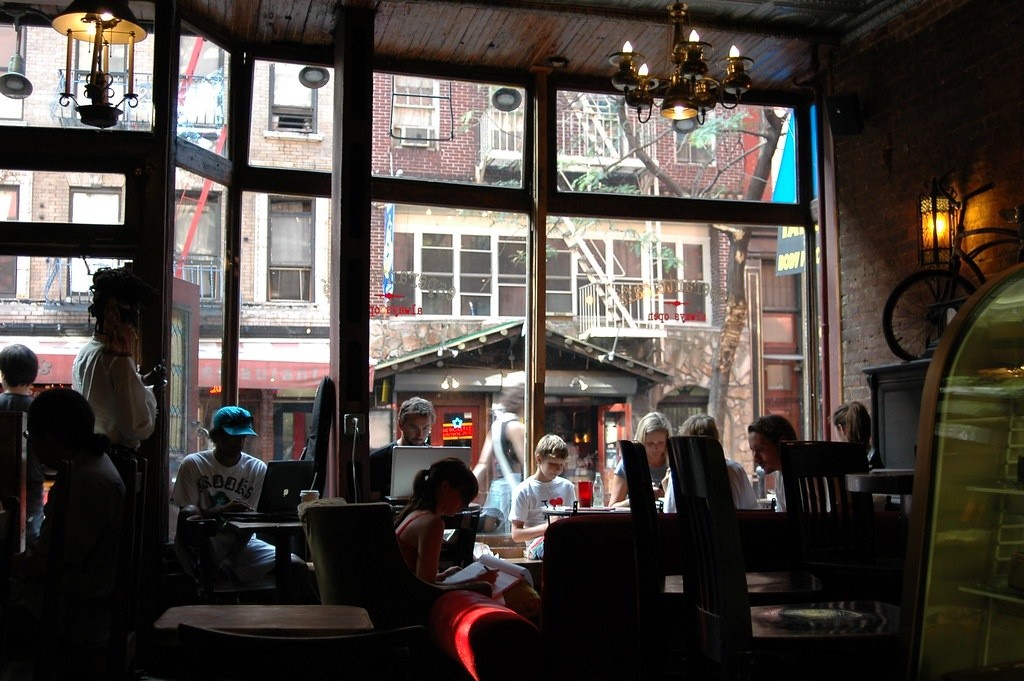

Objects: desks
[153,603,375,681]
[228,522,305,601]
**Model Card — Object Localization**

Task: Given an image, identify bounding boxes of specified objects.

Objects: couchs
[429,511,899,681]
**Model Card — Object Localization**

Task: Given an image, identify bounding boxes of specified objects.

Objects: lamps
[608,0,754,134]
[0,18,33,98]
[912,184,954,265]
[53,1,148,128]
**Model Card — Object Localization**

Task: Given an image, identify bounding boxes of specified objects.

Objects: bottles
[593,472,605,507]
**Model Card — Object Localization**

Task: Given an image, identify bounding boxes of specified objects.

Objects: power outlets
[344,412,366,433]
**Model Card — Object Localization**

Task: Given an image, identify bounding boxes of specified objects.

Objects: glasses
[403,423,433,434]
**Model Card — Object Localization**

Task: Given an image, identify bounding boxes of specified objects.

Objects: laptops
[385,446,472,506]
[222,460,316,519]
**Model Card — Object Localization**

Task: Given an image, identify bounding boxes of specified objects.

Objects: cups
[300,490,320,503]
[578,481,593,508]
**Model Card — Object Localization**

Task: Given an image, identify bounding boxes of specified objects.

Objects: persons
[471,382,580,561]
[393,457,500,587]
[370,396,437,505]
[0,267,157,681]
[826,400,891,512]
[170,406,310,605]
[607,411,761,513]
[747,415,820,512]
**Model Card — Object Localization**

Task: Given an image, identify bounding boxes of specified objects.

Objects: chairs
[186,516,277,603]
[174,622,431,680]
[617,436,915,680]
[34,446,148,681]
[294,498,494,680]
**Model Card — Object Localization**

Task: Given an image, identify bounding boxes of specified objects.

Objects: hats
[214,405,258,436]
